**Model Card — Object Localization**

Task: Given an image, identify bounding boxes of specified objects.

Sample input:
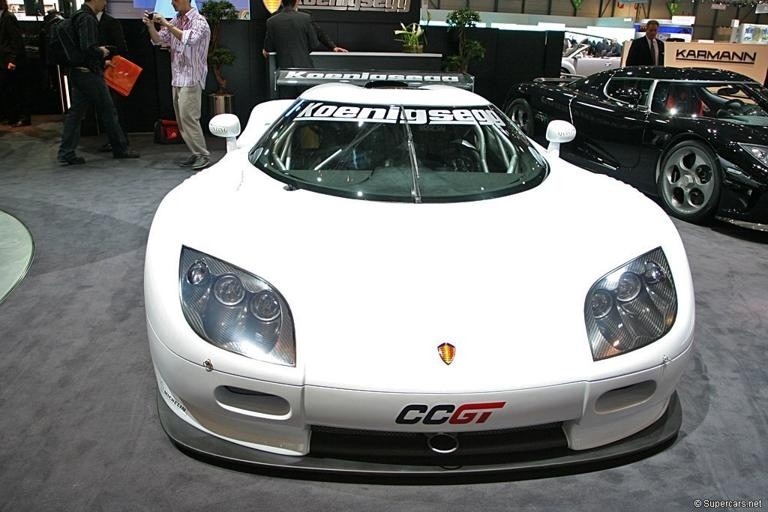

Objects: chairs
[666,88,703,117]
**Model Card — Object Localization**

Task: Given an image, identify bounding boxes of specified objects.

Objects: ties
[651,39,655,65]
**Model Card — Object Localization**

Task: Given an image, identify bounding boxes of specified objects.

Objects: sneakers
[10,117,31,127]
[179,155,209,169]
[114,150,139,159]
[59,154,84,164]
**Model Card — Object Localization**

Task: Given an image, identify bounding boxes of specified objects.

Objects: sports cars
[559,42,626,80]
[496,65,767,232]
[133,76,700,486]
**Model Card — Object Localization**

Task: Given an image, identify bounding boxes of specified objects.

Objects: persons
[84,1,131,152]
[264,0,322,99]
[625,20,665,66]
[56,1,140,166]
[261,1,349,60]
[0,0,32,128]
[606,38,622,57]
[142,0,213,171]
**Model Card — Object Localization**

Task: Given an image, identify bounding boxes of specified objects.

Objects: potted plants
[196,0,240,119]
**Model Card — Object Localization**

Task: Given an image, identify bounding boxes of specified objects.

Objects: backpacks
[46,11,88,68]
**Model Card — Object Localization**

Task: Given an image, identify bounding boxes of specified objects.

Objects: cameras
[146,12,157,20]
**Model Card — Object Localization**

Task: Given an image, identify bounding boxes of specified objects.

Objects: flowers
[393,10,432,53]
